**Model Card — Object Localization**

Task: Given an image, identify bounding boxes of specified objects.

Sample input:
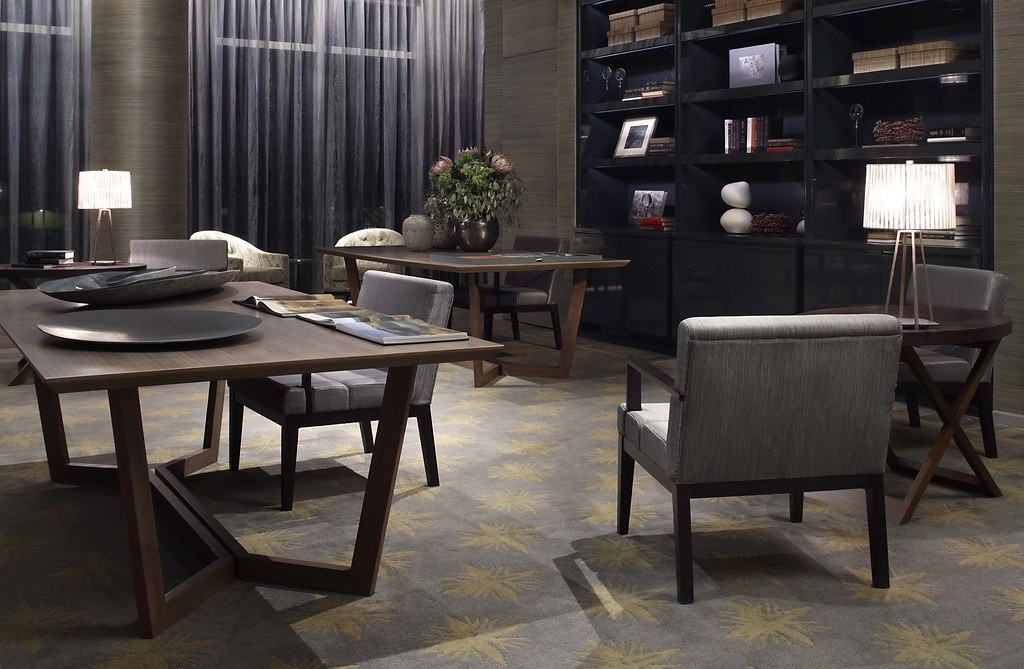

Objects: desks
[311,245,632,383]
[804,304,1014,526]
[0,280,507,639]
[0,262,148,387]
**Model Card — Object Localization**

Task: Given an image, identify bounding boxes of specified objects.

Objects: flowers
[424,146,529,233]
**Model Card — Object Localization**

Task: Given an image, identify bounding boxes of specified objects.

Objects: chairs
[189,231,291,288]
[893,263,1010,458]
[457,236,570,350]
[321,228,407,297]
[616,313,902,604]
[230,269,454,512]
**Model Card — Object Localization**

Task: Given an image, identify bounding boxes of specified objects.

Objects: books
[723,115,804,154]
[233,294,360,318]
[296,308,469,346]
[10,249,74,270]
[867,215,980,249]
[649,137,676,155]
[926,126,983,142]
[639,216,676,232]
[622,79,674,101]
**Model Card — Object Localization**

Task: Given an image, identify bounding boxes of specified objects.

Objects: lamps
[76,168,132,266]
[862,159,956,328]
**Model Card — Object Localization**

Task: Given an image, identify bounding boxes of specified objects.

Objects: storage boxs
[715,0,748,8]
[607,26,636,46]
[850,48,900,73]
[638,4,676,24]
[608,10,639,31]
[897,40,981,69]
[711,2,746,27]
[635,21,674,42]
[746,0,804,21]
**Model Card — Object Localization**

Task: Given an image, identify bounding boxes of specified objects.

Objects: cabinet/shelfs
[575,0,996,419]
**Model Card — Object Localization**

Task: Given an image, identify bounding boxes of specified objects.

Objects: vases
[459,213,500,253]
[401,214,435,250]
[428,216,454,251]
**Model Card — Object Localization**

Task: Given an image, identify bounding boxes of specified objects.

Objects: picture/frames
[614,117,659,156]
